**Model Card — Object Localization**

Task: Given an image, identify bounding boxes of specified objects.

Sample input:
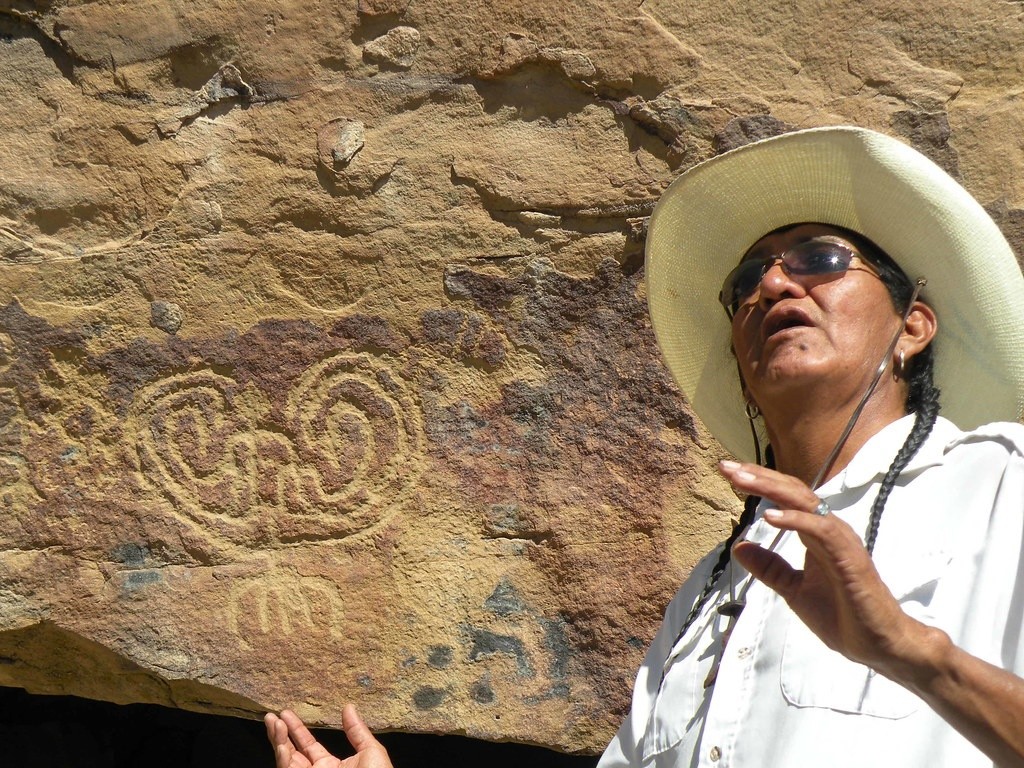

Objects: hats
[644,125,1024,469]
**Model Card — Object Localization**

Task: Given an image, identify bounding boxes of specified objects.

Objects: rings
[815,499,832,517]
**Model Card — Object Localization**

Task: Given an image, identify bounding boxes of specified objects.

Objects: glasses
[717,242,913,326]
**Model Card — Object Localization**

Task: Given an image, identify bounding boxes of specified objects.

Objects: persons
[263,124,1024,768]
